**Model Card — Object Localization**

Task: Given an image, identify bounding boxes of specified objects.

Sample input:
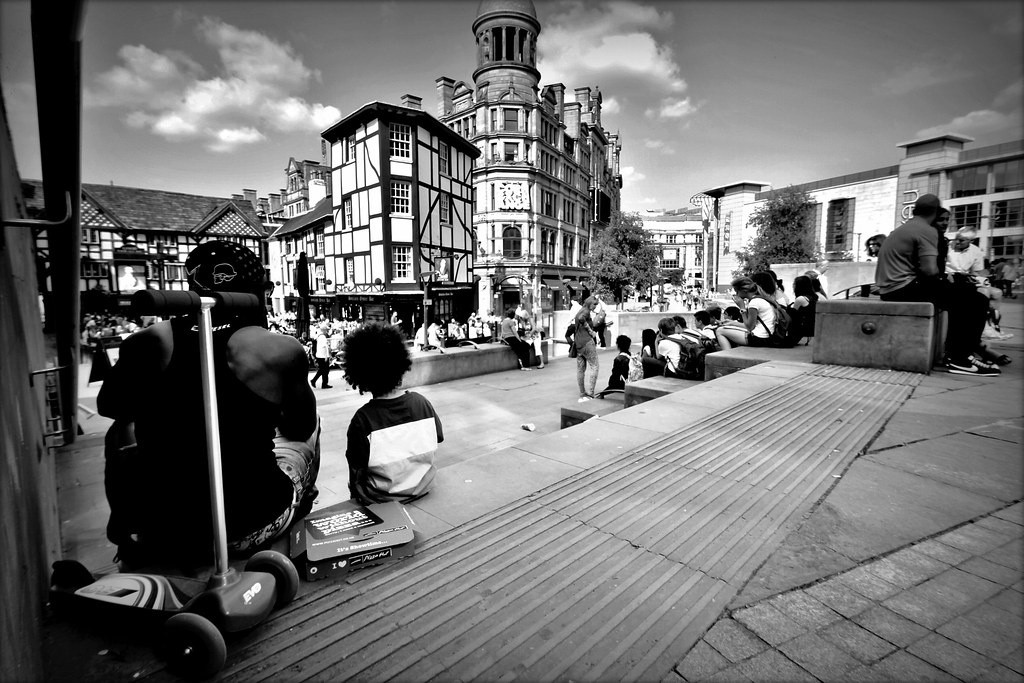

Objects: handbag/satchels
[312,334,323,359]
[568,341,577,358]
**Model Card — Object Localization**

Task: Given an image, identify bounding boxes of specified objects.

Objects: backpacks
[682,329,722,353]
[620,351,644,383]
[749,295,792,348]
[662,336,706,381]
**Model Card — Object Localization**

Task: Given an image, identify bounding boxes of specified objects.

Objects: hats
[185,239,266,301]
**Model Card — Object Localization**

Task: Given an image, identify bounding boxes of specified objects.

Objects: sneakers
[945,355,1001,376]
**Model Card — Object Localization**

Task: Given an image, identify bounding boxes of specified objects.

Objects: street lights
[649,270,654,309]
[847,231,861,261]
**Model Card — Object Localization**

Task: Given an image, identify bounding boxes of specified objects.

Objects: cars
[852,251,878,262]
[683,285,701,294]
[646,282,681,295]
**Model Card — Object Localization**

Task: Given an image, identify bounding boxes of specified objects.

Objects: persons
[865,194,1017,377]
[566,259,829,403]
[61,240,544,578]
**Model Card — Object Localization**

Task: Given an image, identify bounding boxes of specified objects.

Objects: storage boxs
[289,501,423,583]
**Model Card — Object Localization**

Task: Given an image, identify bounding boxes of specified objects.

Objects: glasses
[591,303,597,305]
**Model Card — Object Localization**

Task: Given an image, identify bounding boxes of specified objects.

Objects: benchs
[813,283,948,375]
[398,338,549,390]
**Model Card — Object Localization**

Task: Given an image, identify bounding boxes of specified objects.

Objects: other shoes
[521,367,532,370]
[974,344,1012,366]
[537,365,544,368]
[311,380,316,388]
[322,384,333,389]
[578,396,593,402]
[518,359,523,367]
[981,322,1014,340]
